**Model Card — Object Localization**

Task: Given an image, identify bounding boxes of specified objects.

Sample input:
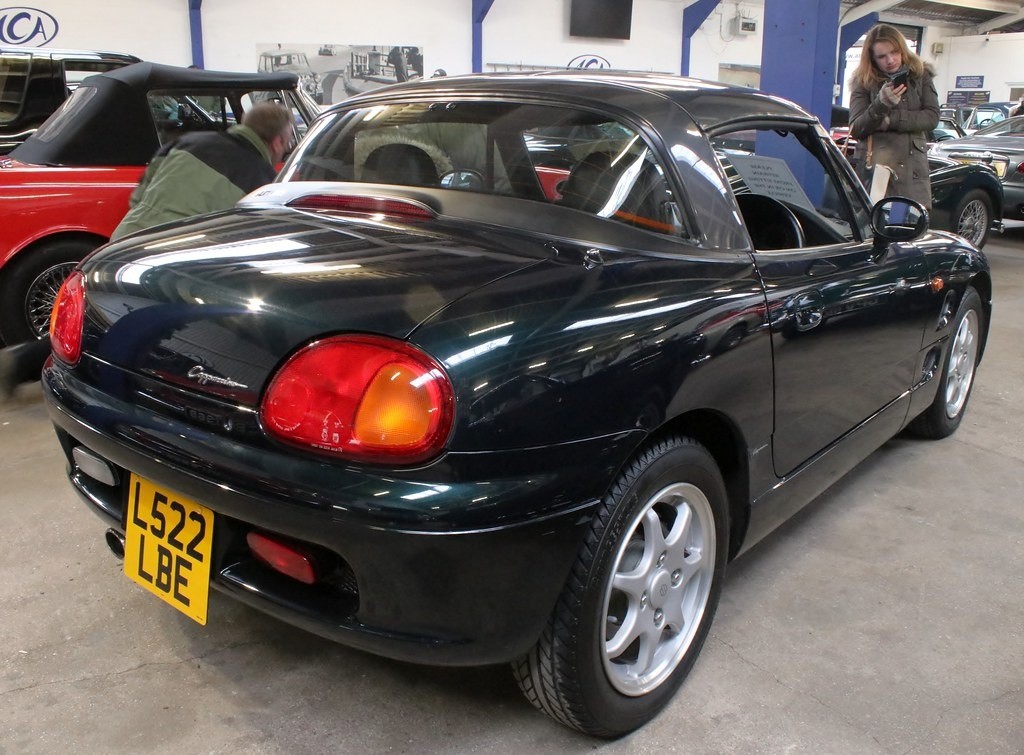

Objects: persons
[407,47,423,78]
[1010,99,1024,134]
[109,103,290,242]
[188,65,226,116]
[849,23,941,211]
[387,46,408,83]
[431,70,446,78]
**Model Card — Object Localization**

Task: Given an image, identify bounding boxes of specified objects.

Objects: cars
[0,63,575,386]
[840,148,1003,248]
[37,72,988,737]
[819,96,1024,235]
[0,47,215,159]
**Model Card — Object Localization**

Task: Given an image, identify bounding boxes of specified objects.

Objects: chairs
[554,150,674,237]
[361,143,440,188]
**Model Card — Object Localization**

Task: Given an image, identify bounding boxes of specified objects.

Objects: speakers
[933,42,943,54]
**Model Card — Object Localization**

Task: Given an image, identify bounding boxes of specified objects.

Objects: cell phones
[891,70,909,95]
